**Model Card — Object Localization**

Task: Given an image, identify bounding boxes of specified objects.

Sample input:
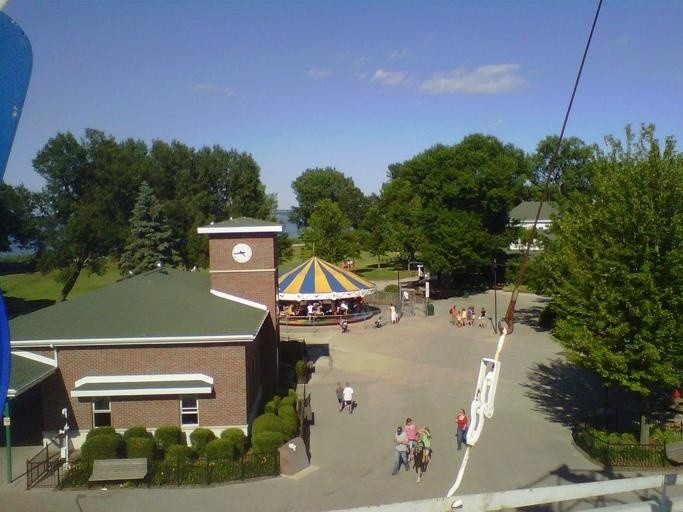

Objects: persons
[404,417,416,462]
[455,409,469,450]
[307,298,362,332]
[417,427,432,465]
[449,304,475,328]
[410,432,425,484]
[377,317,382,327]
[391,427,410,475]
[335,382,344,411]
[403,291,408,300]
[343,382,354,413]
[671,390,680,407]
[347,258,352,268]
[479,307,487,328]
[388,303,401,325]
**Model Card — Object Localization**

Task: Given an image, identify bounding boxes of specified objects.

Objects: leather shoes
[392,455,421,482]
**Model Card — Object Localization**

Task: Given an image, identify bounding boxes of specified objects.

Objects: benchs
[88,458,148,488]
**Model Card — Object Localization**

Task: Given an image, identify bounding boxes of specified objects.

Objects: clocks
[231,243,252,263]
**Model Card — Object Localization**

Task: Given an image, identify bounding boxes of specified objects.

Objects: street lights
[492,259,498,335]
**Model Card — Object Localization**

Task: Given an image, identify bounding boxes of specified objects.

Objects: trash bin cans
[464,291,469,298]
[280,436,309,475]
[426,304,434,316]
